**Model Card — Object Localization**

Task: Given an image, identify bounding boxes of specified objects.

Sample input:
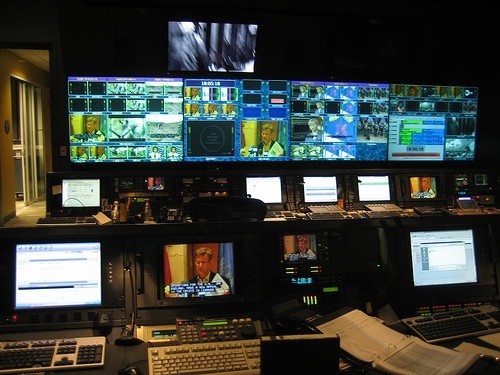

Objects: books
[319,308,477,375]
[259,333,340,375]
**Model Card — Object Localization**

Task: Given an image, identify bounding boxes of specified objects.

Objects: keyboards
[0,336,106,373]
[147,338,260,375]
[36,217,98,226]
[401,304,500,343]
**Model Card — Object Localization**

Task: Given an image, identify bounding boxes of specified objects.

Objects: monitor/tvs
[282,234,317,262]
[15,242,102,308]
[404,222,480,290]
[163,241,236,299]
[45,21,495,217]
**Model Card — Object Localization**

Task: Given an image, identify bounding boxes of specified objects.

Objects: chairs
[259,333,340,375]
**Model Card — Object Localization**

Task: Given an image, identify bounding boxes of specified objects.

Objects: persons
[298,86,324,98]
[191,89,201,100]
[395,102,404,111]
[77,145,107,159]
[151,146,178,159]
[227,105,234,115]
[396,86,417,98]
[360,87,388,140]
[192,106,200,116]
[419,178,435,196]
[293,238,316,259]
[84,117,104,141]
[208,106,216,114]
[316,102,323,113]
[258,124,285,156]
[189,247,231,296]
[305,118,322,141]
[151,177,164,189]
